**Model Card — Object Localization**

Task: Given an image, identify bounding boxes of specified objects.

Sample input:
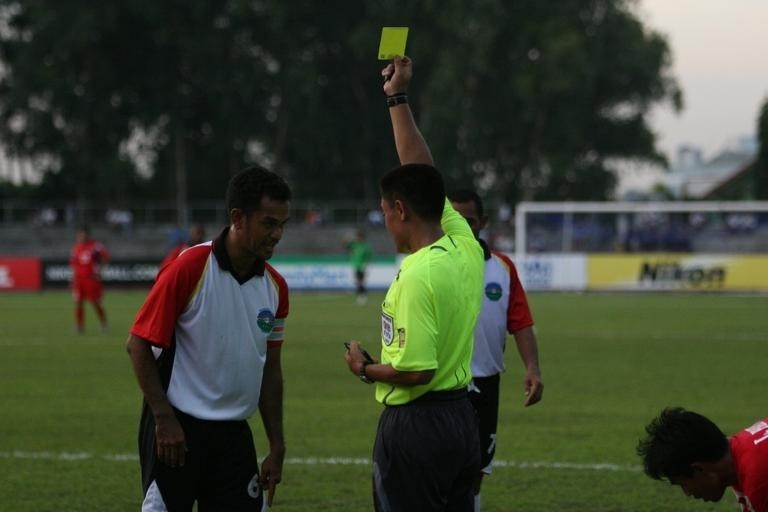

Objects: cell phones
[343,342,375,363]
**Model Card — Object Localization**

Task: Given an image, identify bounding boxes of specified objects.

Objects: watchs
[359,358,376,385]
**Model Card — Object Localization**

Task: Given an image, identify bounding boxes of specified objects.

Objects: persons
[640,406,768,510]
[126,169,293,511]
[70,225,109,335]
[445,184,540,511]
[348,231,370,304]
[343,52,488,509]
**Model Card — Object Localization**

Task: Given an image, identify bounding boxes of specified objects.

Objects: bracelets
[386,92,408,108]
